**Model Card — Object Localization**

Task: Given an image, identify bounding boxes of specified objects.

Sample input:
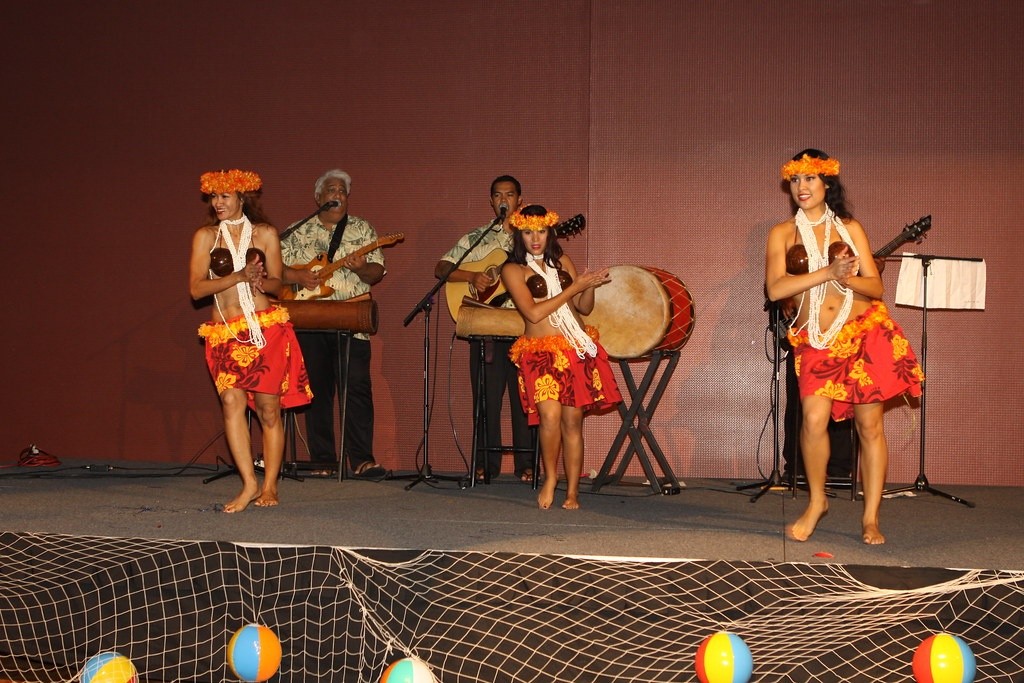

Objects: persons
[435,175,542,482]
[779,349,855,489]
[500,204,623,510]
[188,170,316,514]
[765,149,926,542]
[278,167,388,482]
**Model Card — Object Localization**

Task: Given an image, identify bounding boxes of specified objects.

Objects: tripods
[202,204,329,485]
[388,215,500,492]
[738,300,837,504]
[856,255,984,508]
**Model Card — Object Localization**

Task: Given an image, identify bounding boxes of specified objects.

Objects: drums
[575,263,698,362]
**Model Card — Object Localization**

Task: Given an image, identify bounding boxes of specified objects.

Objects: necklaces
[788,203,861,351]
[209,213,267,348]
[524,252,598,359]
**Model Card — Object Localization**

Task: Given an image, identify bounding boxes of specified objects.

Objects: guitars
[444,214,587,324]
[278,230,407,303]
[769,213,933,351]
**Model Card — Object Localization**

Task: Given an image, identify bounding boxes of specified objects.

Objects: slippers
[352,460,387,478]
[474,469,493,483]
[311,469,332,476]
[521,472,533,484]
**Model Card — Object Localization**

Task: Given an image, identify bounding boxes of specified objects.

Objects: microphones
[327,200,342,207]
[499,203,508,220]
